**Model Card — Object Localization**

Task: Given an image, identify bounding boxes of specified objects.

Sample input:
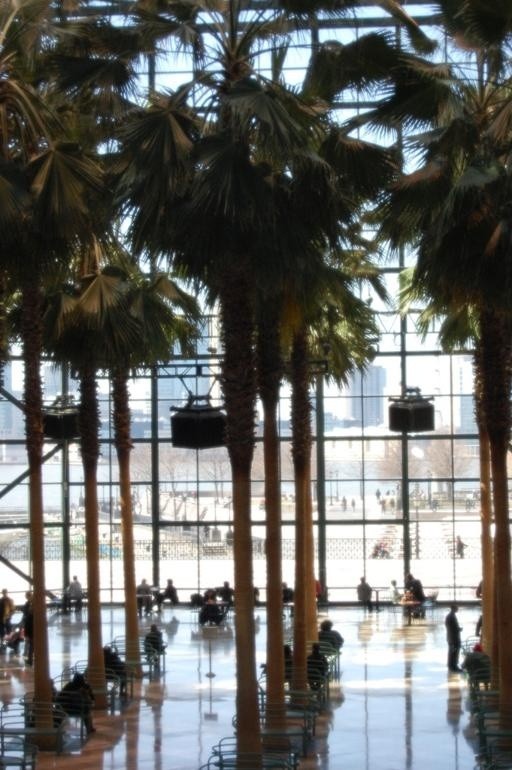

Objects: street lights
[412,495,422,559]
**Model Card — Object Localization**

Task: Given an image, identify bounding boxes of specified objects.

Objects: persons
[318,619,345,657]
[66,575,85,614]
[308,643,330,691]
[144,579,179,612]
[475,581,485,637]
[83,646,127,696]
[54,672,97,734]
[404,573,427,620]
[1,588,33,666]
[390,580,402,603]
[145,624,164,670]
[340,487,395,514]
[443,601,465,674]
[27,687,66,729]
[282,581,295,619]
[315,580,322,603]
[216,581,235,618]
[358,576,374,614]
[120,579,153,612]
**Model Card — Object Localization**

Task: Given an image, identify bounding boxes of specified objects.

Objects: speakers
[46,411,81,438]
[170,412,229,449]
[390,402,436,432]
